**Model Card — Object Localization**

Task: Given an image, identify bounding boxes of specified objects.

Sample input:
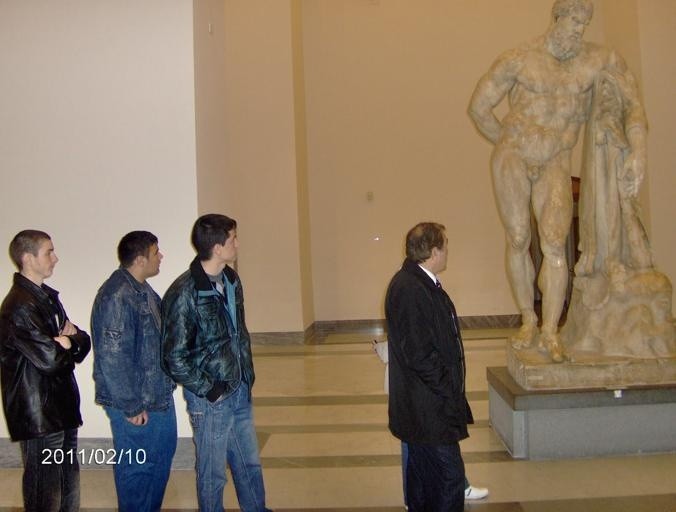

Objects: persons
[382,219,475,510]
[88,229,181,510]
[400,439,491,511]
[465,1,654,365]
[159,212,271,511]
[0,227,91,511]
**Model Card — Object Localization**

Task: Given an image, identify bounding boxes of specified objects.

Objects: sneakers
[463,485,490,500]
